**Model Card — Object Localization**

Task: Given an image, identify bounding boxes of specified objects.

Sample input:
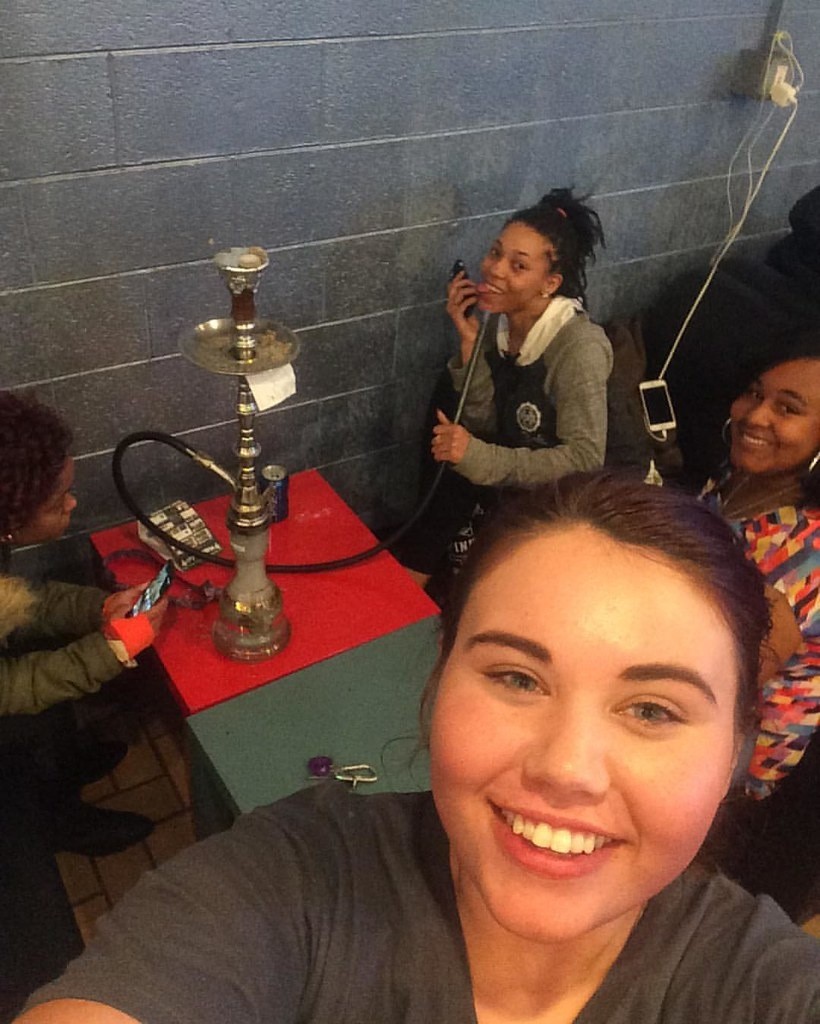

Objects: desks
[183,612,446,841]
[89,468,442,713]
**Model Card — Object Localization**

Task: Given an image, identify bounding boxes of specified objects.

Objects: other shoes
[51,795,155,856]
[49,740,128,785]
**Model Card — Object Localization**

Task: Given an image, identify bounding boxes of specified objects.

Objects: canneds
[260,465,289,523]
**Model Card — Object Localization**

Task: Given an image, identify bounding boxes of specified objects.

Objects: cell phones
[639,380,676,432]
[453,259,474,318]
[125,559,175,619]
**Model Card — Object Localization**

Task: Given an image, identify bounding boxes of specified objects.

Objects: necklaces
[719,475,803,517]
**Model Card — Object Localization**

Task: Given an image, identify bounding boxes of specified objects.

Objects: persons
[0,468,820,1024]
[0,394,181,857]
[683,333,820,926]
[371,188,615,607]
